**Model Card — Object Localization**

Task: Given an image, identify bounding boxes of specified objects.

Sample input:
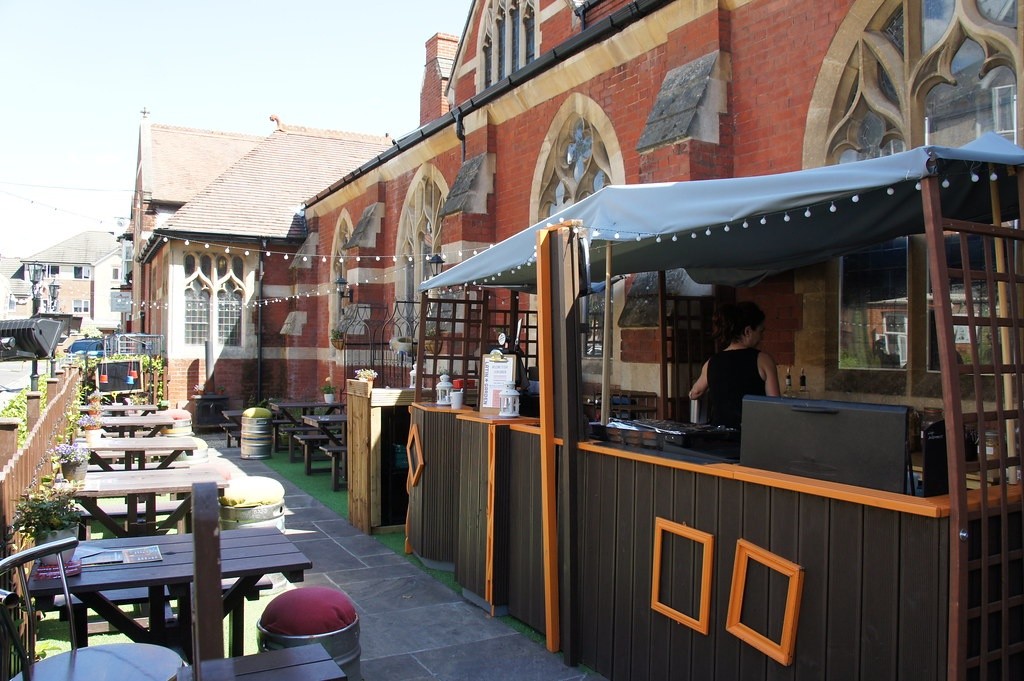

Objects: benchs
[228,431,241,442]
[96,450,173,462]
[52,574,274,658]
[281,425,342,464]
[179,641,347,681]
[320,446,347,491]
[72,499,192,541]
[293,434,342,476]
[219,423,241,448]
[273,419,330,453]
[106,432,161,437]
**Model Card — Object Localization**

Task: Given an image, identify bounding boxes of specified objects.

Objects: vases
[197,391,207,395]
[89,399,100,403]
[60,461,88,482]
[34,522,79,564]
[130,371,138,378]
[86,429,101,443]
[218,390,225,395]
[126,376,134,385]
[100,375,108,383]
[89,410,101,416]
[85,425,100,429]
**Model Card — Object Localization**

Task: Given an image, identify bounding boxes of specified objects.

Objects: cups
[449,391,464,411]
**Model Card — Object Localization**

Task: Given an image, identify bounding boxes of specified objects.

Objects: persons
[688,301,781,429]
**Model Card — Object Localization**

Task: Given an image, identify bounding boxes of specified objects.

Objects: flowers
[216,383,226,390]
[48,436,92,464]
[77,413,105,426]
[194,383,208,392]
[87,402,105,414]
[355,368,378,381]
[87,390,103,400]
[8,473,87,539]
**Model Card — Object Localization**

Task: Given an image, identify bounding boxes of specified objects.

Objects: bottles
[797,366,810,400]
[782,367,797,399]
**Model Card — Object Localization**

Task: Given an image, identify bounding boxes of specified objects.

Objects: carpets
[33,492,272,658]
[262,450,599,677]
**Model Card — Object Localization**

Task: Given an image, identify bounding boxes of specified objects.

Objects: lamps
[498,380,521,418]
[429,253,452,290]
[334,274,354,302]
[0,313,83,391]
[435,374,454,405]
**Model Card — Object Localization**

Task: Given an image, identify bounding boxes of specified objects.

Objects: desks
[101,415,175,437]
[223,408,283,429]
[910,451,1000,490]
[25,525,313,662]
[77,436,198,471]
[79,403,158,431]
[301,414,347,481]
[53,467,230,537]
[270,402,347,456]
[630,418,741,451]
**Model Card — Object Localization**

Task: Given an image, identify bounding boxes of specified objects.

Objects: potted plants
[425,330,443,354]
[320,384,338,403]
[328,329,344,350]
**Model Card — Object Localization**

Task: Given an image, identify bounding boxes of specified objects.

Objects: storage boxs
[453,380,475,389]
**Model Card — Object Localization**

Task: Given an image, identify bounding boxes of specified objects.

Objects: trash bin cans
[239,406,274,460]
[218,475,286,535]
[176,436,209,465]
[160,409,194,437]
[254,584,364,681]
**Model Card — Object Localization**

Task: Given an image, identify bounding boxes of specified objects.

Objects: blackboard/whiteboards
[94,358,144,396]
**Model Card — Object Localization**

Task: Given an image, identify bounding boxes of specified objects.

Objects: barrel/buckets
[257,612,364,681]
[219,499,286,536]
[160,418,192,437]
[177,446,208,469]
[241,416,272,459]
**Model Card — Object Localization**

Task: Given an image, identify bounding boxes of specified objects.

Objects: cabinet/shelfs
[191,394,229,434]
